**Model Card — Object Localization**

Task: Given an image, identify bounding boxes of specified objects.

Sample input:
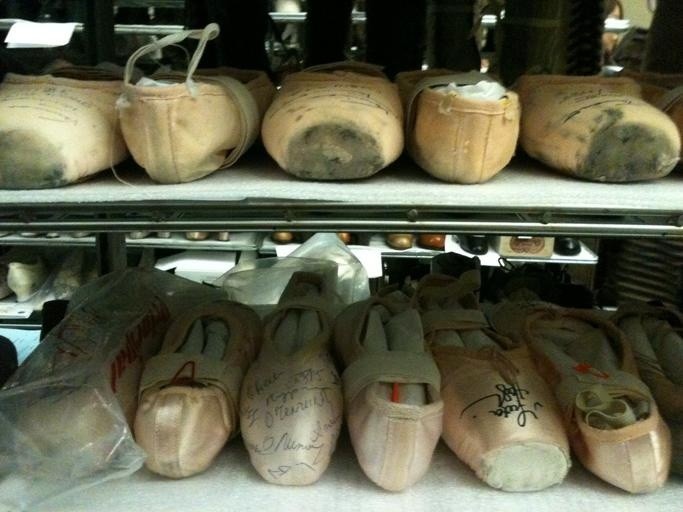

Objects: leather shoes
[385,233,412,248]
[453,233,488,255]
[417,233,445,250]
[273,231,294,242]
[554,237,581,255]
[338,232,356,244]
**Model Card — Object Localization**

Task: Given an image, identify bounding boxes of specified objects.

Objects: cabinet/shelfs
[2,0,681,512]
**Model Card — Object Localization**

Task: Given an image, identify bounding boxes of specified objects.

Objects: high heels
[6,257,50,301]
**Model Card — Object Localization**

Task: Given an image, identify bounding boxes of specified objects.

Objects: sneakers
[608,299,683,480]
[262,62,404,181]
[134,298,264,479]
[415,273,571,492]
[0,58,146,189]
[527,302,673,495]
[53,249,88,300]
[240,271,344,486]
[621,68,683,128]
[399,68,521,184]
[1,267,228,511]
[509,75,682,182]
[116,22,276,183]
[330,298,445,492]
